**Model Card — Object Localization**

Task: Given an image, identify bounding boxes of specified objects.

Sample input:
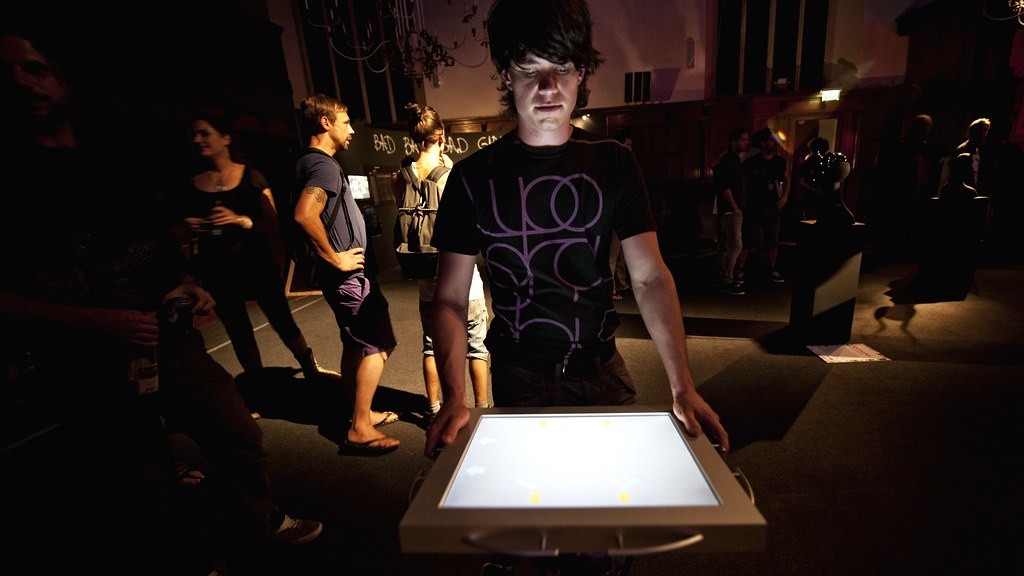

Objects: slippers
[372,411,399,428]
[345,436,399,451]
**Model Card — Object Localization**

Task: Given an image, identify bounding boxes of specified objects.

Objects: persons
[799,137,836,231]
[883,115,934,247]
[1,27,325,576]
[712,127,754,296]
[944,118,995,249]
[388,102,495,432]
[169,105,335,420]
[424,2,733,463]
[283,93,409,455]
[598,129,643,306]
[744,126,791,285]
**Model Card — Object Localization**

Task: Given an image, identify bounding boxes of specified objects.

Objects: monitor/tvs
[400,403,769,556]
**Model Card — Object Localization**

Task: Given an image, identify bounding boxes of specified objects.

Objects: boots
[295,347,343,383]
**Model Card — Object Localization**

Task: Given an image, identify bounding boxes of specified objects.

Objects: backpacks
[392,164,452,281]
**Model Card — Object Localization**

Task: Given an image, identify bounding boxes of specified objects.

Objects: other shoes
[734,274,745,284]
[720,277,744,295]
[423,402,443,431]
[771,271,785,282]
[269,516,324,543]
